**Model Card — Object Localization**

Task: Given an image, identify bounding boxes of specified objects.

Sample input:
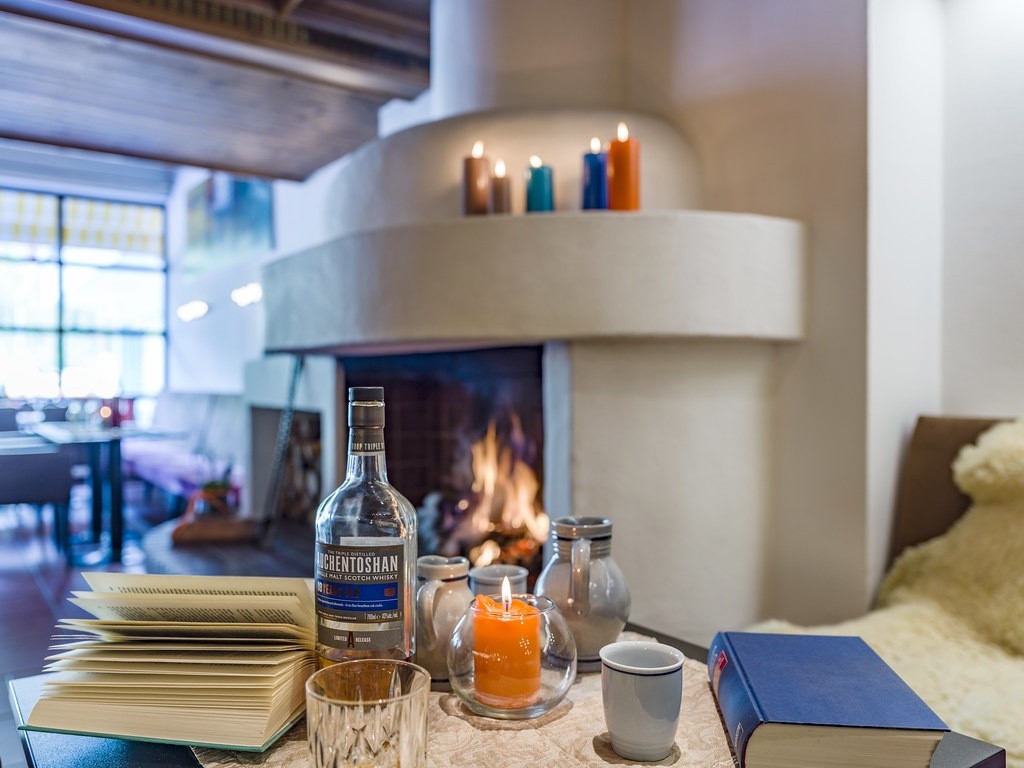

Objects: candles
[464,139,510,215]
[583,122,640,211]
[523,155,554,212]
[471,575,542,708]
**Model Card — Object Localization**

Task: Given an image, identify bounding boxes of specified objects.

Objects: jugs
[405,554,476,691]
[532,515,631,672]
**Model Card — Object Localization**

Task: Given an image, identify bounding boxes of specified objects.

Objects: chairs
[116,391,248,517]
[0,407,71,553]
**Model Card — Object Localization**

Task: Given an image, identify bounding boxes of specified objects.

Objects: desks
[33,420,193,570]
[9,620,1007,768]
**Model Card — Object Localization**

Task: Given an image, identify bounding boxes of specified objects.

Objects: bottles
[314,387,418,710]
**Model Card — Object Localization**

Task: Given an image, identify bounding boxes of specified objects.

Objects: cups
[470,563,530,600]
[599,640,685,762]
[304,658,432,768]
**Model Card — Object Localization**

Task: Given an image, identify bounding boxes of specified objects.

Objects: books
[707,630,951,767]
[21,571,318,750]
